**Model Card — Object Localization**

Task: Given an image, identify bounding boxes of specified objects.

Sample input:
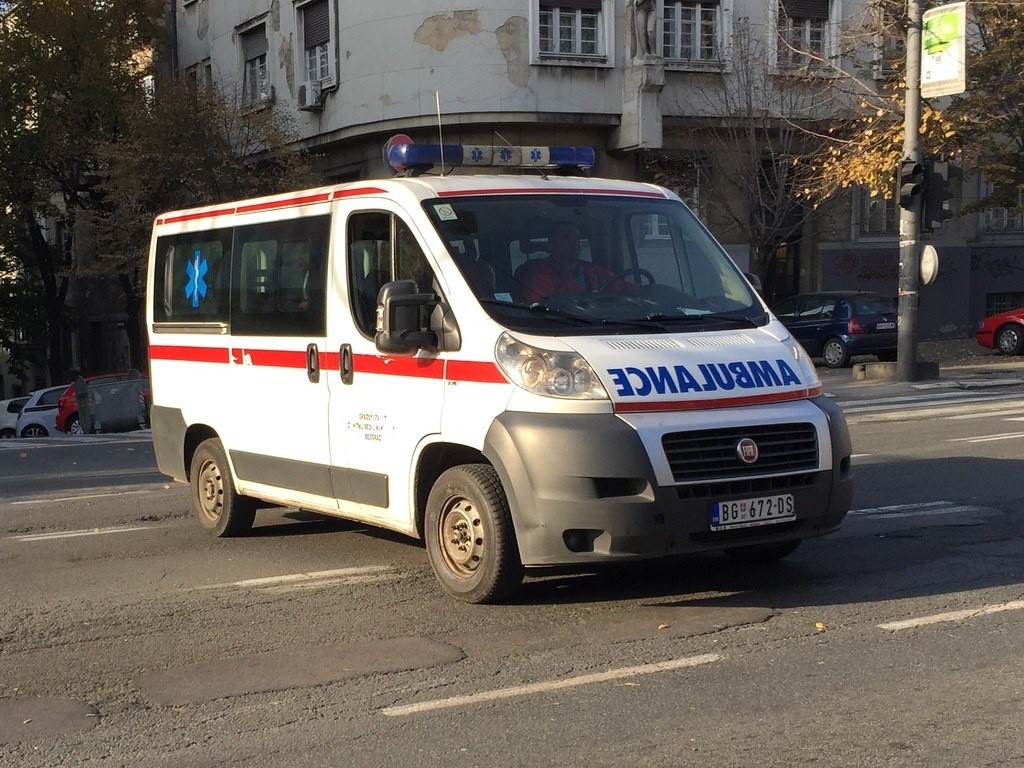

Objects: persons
[515,220,642,308]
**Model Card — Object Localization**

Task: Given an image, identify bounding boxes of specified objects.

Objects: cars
[16,383,119,438]
[0,391,60,440]
[976,307,1024,355]
[56,371,152,436]
[768,289,896,368]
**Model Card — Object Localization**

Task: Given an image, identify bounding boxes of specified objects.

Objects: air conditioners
[298,80,322,111]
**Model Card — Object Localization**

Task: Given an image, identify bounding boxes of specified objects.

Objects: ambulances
[143,134,856,608]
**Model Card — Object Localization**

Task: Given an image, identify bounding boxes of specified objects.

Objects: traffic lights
[896,159,923,206]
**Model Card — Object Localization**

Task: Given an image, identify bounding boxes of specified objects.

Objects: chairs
[513,259,543,305]
[364,269,392,329]
[248,270,284,316]
